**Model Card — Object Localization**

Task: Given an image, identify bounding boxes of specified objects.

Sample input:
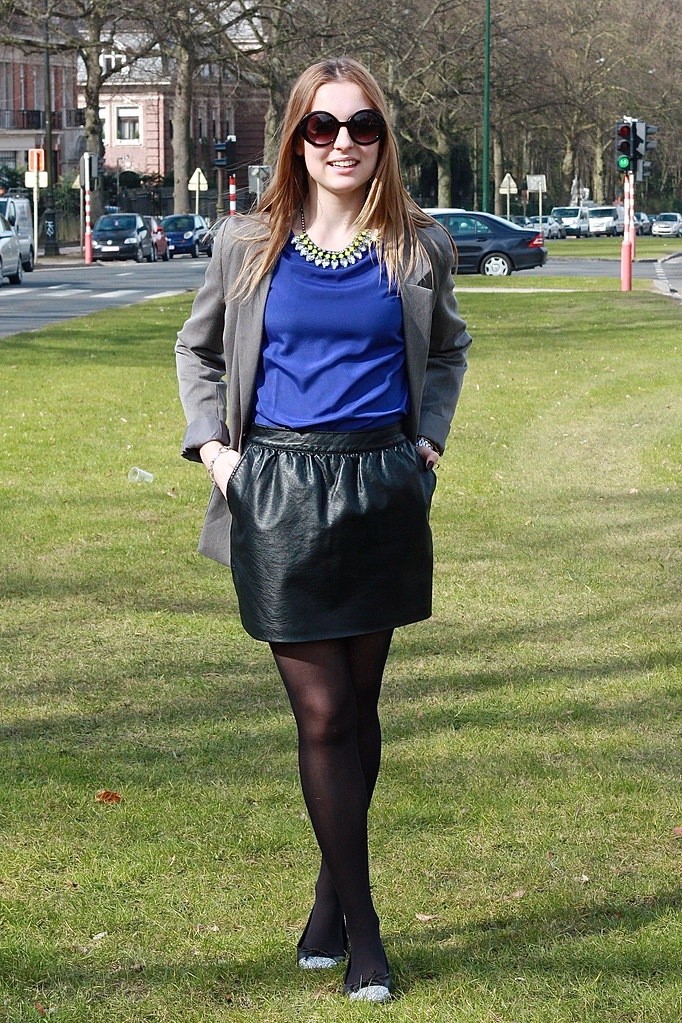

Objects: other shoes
[297,903,349,970]
[343,944,394,1002]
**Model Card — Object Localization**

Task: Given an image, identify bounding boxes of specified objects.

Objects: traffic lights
[615,121,634,172]
[90,155,107,190]
[635,123,660,182]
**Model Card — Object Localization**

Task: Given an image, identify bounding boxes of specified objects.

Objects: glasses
[296,108,386,148]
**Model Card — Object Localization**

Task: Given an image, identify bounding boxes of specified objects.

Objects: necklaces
[291,209,375,270]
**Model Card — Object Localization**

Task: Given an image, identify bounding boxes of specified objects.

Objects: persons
[176,59,471,1003]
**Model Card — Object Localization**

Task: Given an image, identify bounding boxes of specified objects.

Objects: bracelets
[208,446,230,482]
[416,438,433,450]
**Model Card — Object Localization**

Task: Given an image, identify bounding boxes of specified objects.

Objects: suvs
[0,188,35,272]
[91,213,155,263]
[552,207,591,238]
[588,206,642,237]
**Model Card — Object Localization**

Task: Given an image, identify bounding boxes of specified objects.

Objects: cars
[500,215,566,240]
[159,213,214,258]
[0,213,22,285]
[635,212,682,238]
[419,208,548,276]
[144,216,170,262]
